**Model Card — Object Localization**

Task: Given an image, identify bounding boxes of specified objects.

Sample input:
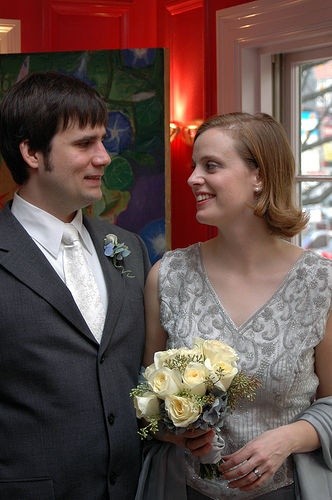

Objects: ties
[62,225,106,345]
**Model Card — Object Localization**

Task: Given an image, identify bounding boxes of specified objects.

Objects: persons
[134,112,332,500]
[0,71,153,500]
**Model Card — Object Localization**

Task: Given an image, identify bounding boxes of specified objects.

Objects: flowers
[103,234,136,279]
[129,339,262,481]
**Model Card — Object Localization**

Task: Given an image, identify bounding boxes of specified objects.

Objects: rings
[253,468,262,478]
[185,439,192,456]
